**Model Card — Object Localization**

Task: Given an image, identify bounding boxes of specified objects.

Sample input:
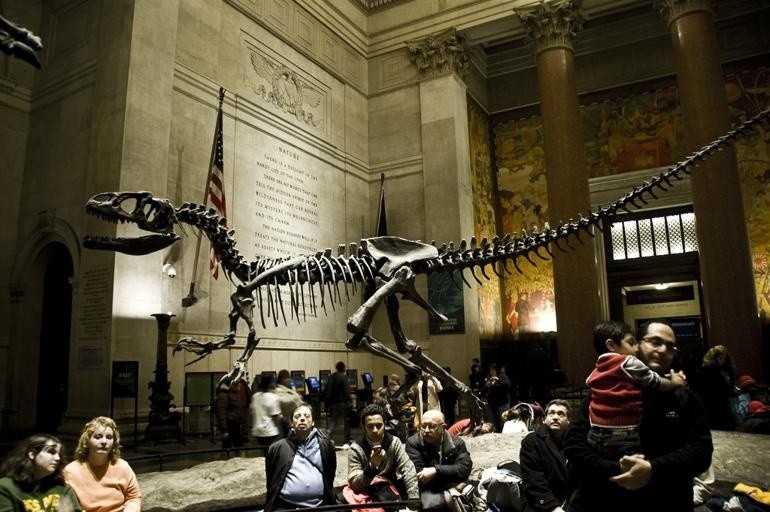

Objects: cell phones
[372,445,382,454]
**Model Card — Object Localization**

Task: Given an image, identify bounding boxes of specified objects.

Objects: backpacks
[486,479,523,508]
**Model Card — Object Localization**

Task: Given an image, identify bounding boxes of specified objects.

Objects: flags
[200,102,231,283]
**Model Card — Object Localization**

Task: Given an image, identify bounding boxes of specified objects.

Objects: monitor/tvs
[363,372,373,383]
[308,377,320,389]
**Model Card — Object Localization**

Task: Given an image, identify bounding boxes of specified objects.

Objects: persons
[62,414,141,512]
[564,318,715,512]
[216,370,306,449]
[379,366,461,438]
[323,361,354,445]
[519,398,575,512]
[262,402,336,511]
[514,292,536,330]
[565,320,688,512]
[469,359,510,433]
[346,404,420,512]
[731,374,756,423]
[691,343,739,432]
[744,400,770,419]
[404,409,472,490]
[0,432,83,512]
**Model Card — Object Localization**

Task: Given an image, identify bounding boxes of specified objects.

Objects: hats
[738,375,756,389]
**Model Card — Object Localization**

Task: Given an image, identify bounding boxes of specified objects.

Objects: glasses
[643,336,679,358]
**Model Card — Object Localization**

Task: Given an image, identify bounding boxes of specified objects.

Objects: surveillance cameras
[167,265,176,279]
